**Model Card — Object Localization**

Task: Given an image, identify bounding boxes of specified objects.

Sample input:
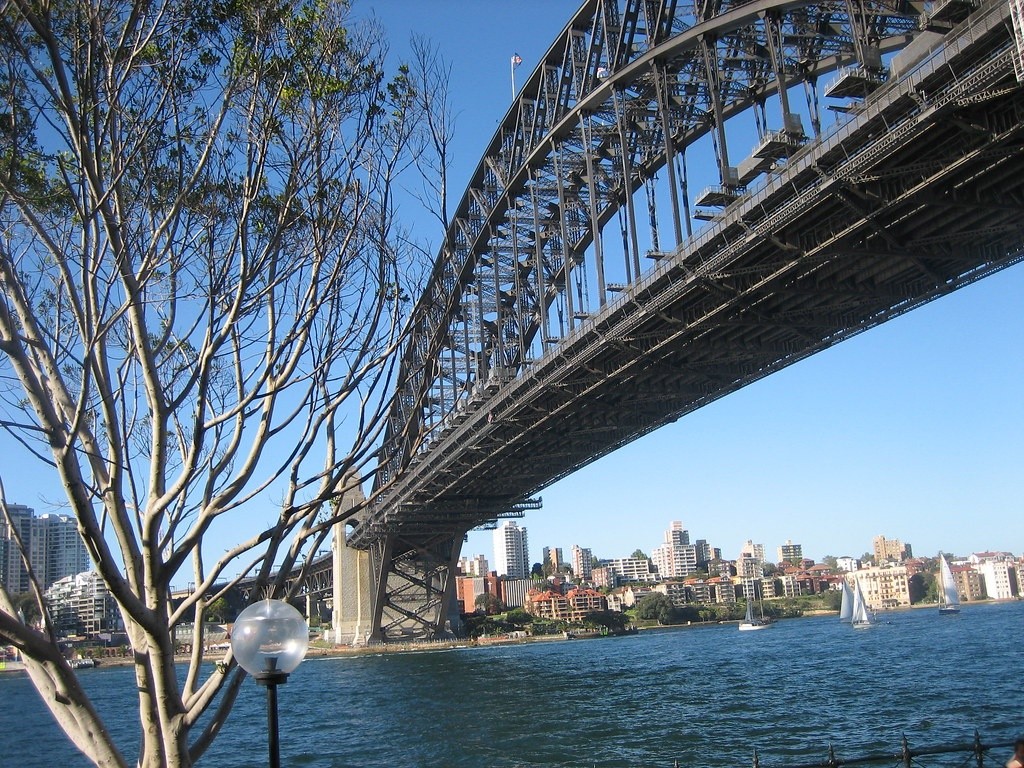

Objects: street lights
[230,598,310,768]
[510,51,523,103]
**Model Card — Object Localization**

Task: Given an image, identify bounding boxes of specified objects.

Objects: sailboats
[737,585,779,631]
[838,575,879,629]
[936,551,961,616]
